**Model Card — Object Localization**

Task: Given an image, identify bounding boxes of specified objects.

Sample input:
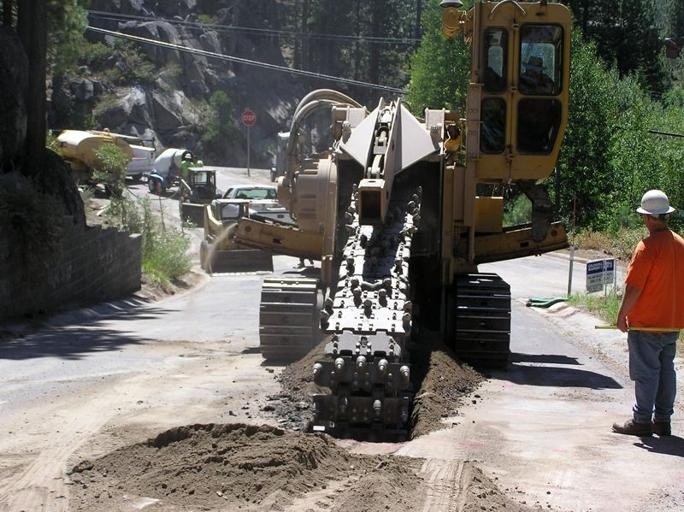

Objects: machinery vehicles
[179,168,221,227]
[233,0,571,442]
[200,199,273,273]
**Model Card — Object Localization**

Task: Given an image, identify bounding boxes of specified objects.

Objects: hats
[523,54,549,73]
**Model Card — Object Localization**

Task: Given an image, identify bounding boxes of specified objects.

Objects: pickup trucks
[222,184,296,224]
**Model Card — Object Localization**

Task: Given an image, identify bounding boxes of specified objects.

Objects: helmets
[637,188,676,217]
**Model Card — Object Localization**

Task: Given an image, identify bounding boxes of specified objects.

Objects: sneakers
[610,417,672,437]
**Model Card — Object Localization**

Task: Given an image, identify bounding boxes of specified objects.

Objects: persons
[512,55,556,154]
[610,188,683,438]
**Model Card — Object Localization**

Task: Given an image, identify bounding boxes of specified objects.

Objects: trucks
[50,128,156,179]
